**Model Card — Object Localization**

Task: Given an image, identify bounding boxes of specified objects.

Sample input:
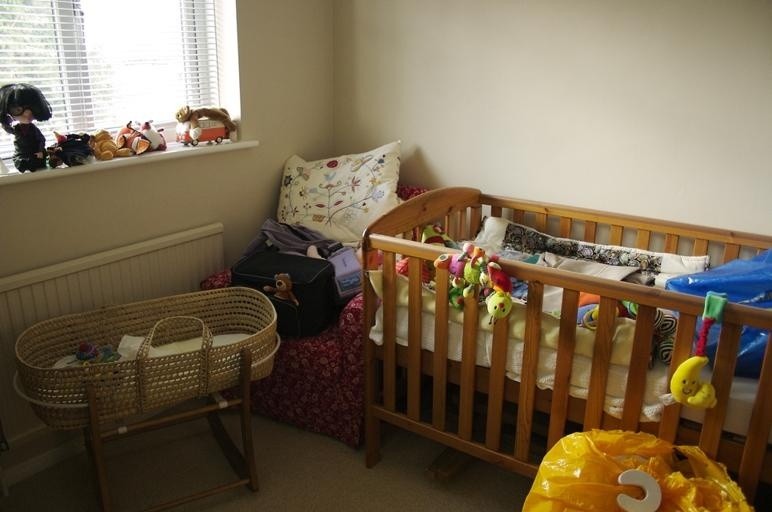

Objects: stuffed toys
[264,273,299,306]
[422,225,512,325]
[0,83,237,172]
[577,291,728,411]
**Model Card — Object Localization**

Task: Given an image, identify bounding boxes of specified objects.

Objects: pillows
[275,137,405,251]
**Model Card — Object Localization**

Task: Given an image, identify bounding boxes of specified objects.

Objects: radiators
[1,220,228,454]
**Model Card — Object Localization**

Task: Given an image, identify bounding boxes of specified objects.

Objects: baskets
[12,284,279,432]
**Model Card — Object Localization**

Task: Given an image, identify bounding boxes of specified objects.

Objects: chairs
[198,181,435,452]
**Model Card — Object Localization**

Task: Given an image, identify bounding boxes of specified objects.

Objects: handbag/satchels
[226,247,343,339]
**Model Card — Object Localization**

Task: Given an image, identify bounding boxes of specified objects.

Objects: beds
[359,184,771,506]
